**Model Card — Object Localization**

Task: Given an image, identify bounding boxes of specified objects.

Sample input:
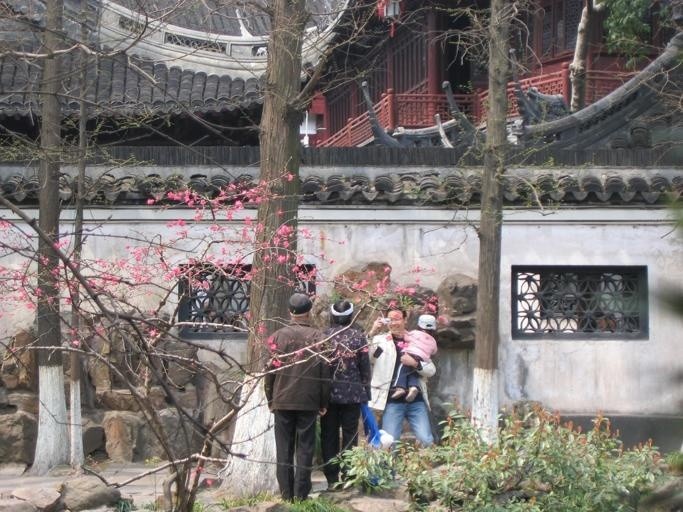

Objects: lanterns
[377,0,405,39]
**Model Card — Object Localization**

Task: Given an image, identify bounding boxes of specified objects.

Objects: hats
[417,314,437,331]
[288,293,313,316]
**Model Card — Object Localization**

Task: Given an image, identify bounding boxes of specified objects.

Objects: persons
[390,314,438,403]
[264,294,329,506]
[318,300,371,490]
[363,304,434,478]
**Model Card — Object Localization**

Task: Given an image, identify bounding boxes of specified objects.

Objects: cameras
[381,317,392,326]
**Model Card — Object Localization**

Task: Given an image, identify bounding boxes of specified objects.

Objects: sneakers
[404,386,419,403]
[391,388,406,401]
